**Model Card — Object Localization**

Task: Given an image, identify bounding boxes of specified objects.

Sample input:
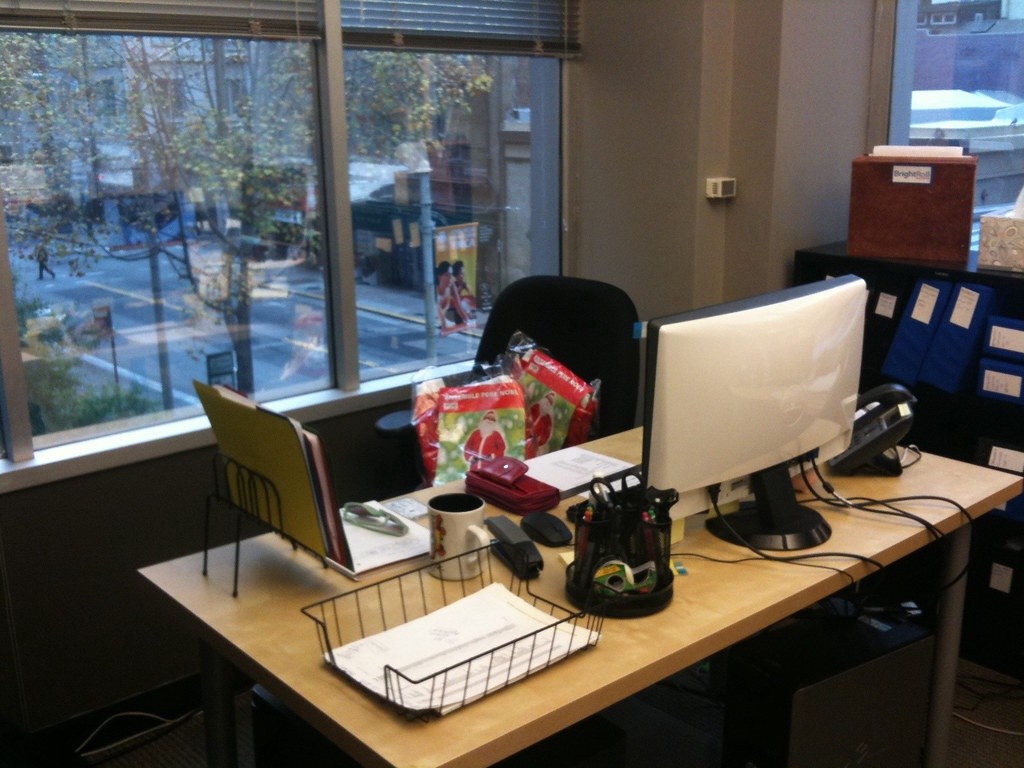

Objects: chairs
[376,274,642,768]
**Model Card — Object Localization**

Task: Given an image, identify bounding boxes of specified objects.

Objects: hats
[545,393,554,405]
[483,412,496,421]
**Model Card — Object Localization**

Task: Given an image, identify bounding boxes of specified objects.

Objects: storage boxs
[846,153,979,262]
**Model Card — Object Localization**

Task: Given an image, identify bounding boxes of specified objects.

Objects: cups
[430,493,490,582]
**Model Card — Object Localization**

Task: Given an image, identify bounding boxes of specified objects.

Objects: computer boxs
[721,599,936,768]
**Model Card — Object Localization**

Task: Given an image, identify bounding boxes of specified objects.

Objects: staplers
[482,514,546,582]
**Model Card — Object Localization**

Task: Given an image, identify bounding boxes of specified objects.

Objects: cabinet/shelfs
[794,239,1024,683]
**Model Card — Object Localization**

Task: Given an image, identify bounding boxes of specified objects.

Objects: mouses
[521,511,572,547]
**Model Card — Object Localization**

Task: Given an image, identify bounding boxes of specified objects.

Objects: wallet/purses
[464,455,560,516]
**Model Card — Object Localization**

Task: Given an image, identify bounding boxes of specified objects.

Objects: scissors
[591,468,646,509]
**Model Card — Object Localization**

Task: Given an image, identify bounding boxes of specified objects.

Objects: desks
[136,425,1024,768]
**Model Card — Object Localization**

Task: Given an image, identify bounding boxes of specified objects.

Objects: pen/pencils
[577,498,604,522]
[640,505,659,524]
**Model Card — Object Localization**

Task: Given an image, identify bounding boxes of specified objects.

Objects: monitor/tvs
[642,274,867,551]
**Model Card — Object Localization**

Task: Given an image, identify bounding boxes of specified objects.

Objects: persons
[435,261,468,329]
[37,242,56,281]
[451,261,477,320]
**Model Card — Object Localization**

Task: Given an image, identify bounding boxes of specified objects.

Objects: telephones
[828,381,922,477]
